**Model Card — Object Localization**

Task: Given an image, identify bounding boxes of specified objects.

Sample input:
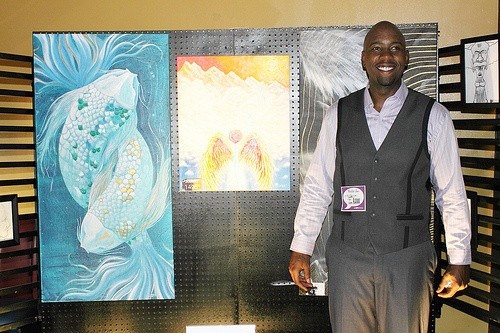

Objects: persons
[288,22,473,333]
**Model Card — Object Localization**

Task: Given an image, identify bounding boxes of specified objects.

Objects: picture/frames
[459,33,499,111]
[0,194,20,248]
